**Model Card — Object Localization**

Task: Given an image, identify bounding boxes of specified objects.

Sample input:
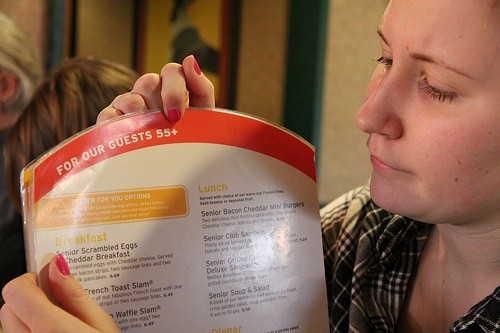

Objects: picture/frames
[0,0,77,70]
[132,0,240,110]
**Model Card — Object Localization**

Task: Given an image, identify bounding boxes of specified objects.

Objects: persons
[0,3,500,333]
[0,16,40,125]
[0,57,141,269]
